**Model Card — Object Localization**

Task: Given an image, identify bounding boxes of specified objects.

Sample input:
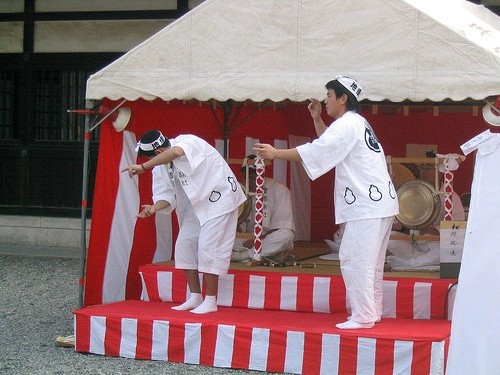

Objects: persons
[324,163,466,271]
[121,130,247,313]
[231,154,295,266]
[252,75,400,329]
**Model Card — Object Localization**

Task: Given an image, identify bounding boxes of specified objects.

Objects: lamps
[482,96,500,128]
[98,105,134,133]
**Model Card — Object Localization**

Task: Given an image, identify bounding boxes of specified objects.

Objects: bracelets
[141,164,147,171]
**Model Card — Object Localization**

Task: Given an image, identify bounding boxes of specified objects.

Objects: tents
[74,0,500,375]
